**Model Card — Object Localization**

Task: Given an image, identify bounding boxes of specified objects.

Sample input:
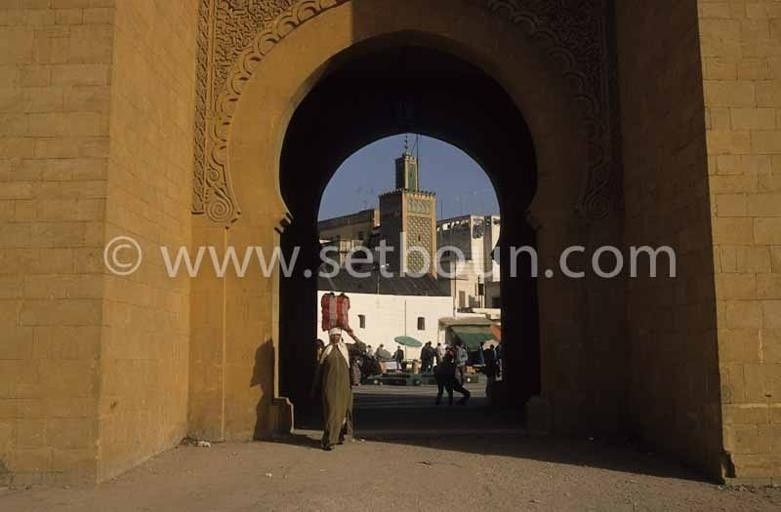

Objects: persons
[308,324,367,451]
[419,341,504,407]
[375,344,385,357]
[393,345,404,370]
[367,345,375,358]
